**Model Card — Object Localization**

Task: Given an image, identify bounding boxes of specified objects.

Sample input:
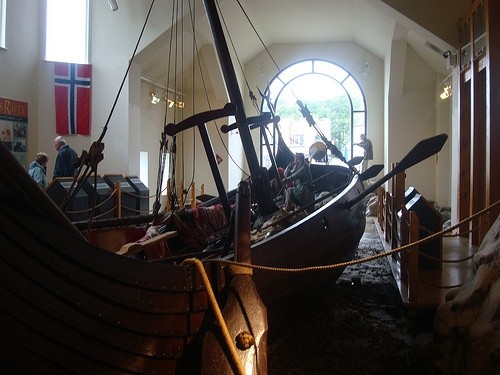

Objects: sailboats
[0,0,450,375]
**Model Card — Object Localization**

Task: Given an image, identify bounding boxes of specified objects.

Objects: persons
[279,153,317,208]
[352,133,377,176]
[28,152,49,192]
[50,136,82,187]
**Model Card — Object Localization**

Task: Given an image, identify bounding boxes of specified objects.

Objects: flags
[55,62,90,136]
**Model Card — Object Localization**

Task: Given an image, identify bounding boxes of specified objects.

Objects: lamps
[107,0,118,11]
[148,89,184,109]
